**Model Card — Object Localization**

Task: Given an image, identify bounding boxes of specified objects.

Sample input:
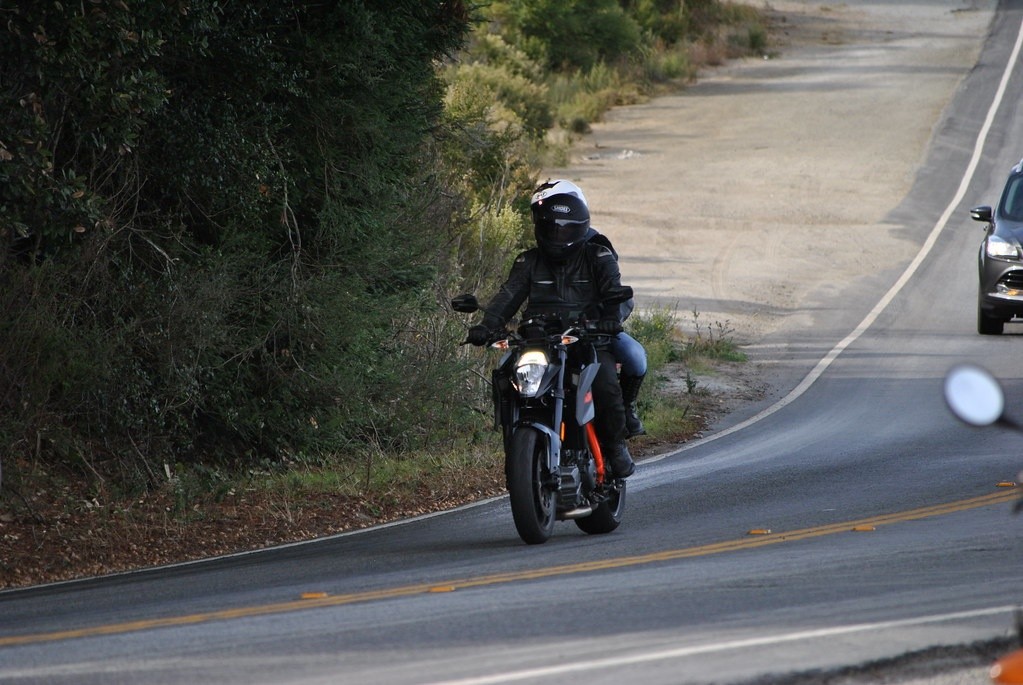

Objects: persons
[466,178,648,479]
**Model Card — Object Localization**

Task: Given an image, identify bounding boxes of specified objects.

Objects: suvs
[970,158,1023,336]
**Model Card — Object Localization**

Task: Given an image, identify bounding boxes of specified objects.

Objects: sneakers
[607,436,636,479]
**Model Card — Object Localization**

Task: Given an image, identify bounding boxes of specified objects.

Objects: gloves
[597,305,623,336]
[467,325,488,346]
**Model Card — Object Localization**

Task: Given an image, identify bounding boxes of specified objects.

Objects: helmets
[533,194,592,269]
[527,178,588,209]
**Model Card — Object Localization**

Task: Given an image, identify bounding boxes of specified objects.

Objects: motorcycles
[452,287,647,544]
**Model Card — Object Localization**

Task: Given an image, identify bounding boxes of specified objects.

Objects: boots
[620,371,647,435]
